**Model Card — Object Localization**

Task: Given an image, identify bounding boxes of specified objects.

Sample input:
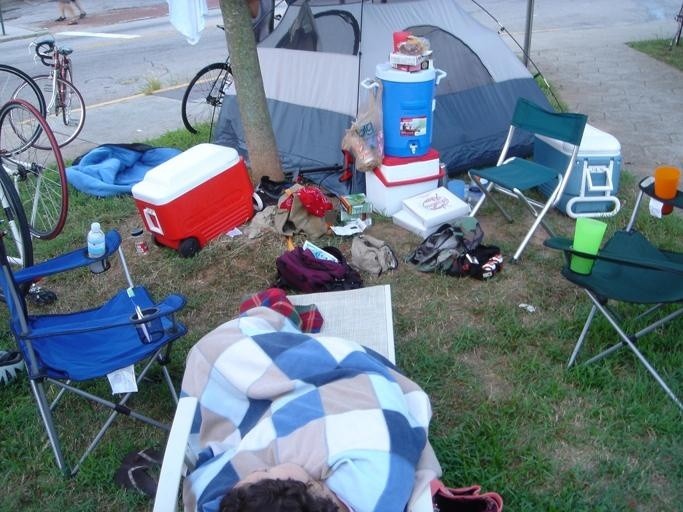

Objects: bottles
[131,228,150,258]
[86,223,105,273]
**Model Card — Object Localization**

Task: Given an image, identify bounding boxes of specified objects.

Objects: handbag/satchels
[271,246,362,294]
[351,235,399,277]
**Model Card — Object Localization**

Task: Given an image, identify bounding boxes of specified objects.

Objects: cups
[654,168,680,216]
[568,215,610,275]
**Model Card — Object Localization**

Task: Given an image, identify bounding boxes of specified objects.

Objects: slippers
[122,444,163,467]
[113,466,157,499]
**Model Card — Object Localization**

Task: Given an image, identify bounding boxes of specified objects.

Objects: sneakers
[55,17,67,22]
[80,11,87,18]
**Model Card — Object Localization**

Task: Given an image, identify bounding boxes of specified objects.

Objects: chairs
[468,98,589,263]
[0,229,181,478]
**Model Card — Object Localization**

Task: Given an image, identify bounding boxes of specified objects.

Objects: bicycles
[8,32,86,150]
[182,0,360,135]
[0,64,69,304]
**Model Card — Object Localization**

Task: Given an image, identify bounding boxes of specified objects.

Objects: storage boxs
[365,149,444,217]
[543,174,683,411]
[132,140,263,256]
[533,124,623,218]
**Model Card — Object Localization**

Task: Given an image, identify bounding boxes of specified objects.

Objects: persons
[224,461,347,512]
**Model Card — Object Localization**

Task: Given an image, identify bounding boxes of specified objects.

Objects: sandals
[67,20,79,25]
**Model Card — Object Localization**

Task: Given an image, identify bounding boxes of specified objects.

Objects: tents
[211,2,570,191]
